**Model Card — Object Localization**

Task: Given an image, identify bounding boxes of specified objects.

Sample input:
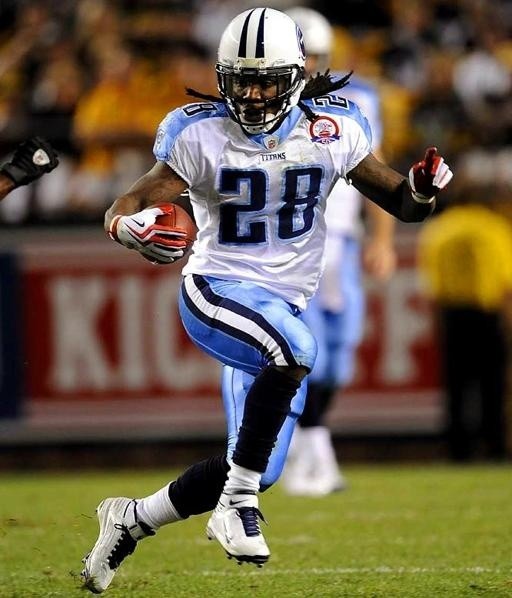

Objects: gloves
[107,205,189,267]
[408,146,454,205]
[1,136,59,188]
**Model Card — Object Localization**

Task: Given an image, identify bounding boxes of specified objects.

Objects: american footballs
[139,202,197,265]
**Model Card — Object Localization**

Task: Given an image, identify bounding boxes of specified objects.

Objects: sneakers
[283,424,346,499]
[205,489,271,569]
[80,496,156,594]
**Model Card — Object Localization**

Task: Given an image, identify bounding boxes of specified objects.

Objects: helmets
[214,7,306,137]
[284,5,334,74]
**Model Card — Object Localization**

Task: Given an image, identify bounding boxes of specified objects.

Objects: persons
[1,1,512,223]
[269,7,385,500]
[407,174,511,465]
[76,5,453,594]
